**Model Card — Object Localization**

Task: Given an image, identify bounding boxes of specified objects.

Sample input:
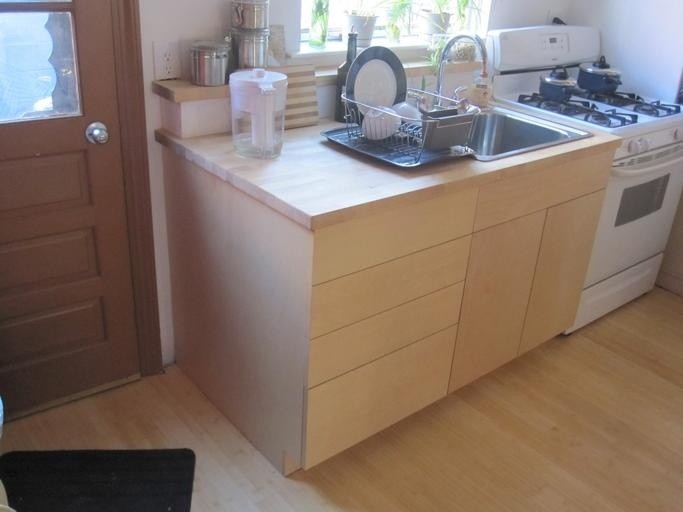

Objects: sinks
[468,106,594,162]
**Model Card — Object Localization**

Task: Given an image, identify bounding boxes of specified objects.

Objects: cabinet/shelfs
[156,130,480,476]
[452,133,620,397]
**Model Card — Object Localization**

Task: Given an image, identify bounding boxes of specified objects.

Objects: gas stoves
[495,89,683,160]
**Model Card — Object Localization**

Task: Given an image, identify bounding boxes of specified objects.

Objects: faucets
[436,31,488,103]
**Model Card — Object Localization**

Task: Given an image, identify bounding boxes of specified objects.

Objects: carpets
[0,447,196,512]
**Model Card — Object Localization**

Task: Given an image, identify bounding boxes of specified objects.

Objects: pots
[578,56,621,92]
[539,65,577,101]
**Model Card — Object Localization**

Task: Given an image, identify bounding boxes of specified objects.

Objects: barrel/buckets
[188,46,228,86]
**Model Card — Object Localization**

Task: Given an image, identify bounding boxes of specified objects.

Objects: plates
[345,44,407,126]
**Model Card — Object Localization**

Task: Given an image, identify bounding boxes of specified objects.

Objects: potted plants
[387,0,482,77]
[342,0,384,49]
[307,1,330,47]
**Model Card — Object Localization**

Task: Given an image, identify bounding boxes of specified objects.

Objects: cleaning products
[469,76,493,104]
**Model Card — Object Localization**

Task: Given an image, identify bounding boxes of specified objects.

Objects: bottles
[337,33,357,122]
[470,73,493,106]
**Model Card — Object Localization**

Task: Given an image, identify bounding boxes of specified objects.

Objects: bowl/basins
[362,106,401,141]
[393,102,421,124]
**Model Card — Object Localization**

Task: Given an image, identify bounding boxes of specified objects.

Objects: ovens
[582,156,683,288]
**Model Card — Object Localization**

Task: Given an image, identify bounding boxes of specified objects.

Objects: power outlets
[150,38,183,81]
